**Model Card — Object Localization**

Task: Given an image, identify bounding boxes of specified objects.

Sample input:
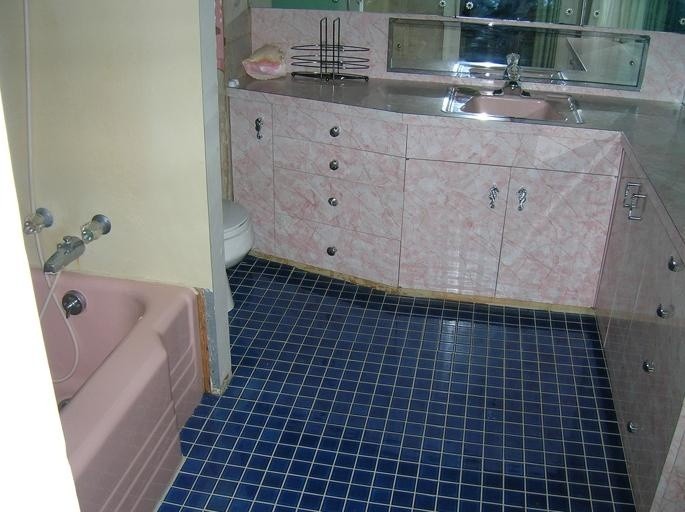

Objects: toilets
[223,197,255,312]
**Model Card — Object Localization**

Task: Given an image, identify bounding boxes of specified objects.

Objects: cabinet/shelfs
[398,112,623,315]
[225,75,408,298]
[592,131,685,511]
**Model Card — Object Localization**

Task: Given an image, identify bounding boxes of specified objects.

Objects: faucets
[508,65,522,90]
[507,53,520,65]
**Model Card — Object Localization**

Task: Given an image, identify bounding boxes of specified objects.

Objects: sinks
[455,60,567,85]
[445,84,585,124]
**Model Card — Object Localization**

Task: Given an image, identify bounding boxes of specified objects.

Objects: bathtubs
[32,272,206,512]
